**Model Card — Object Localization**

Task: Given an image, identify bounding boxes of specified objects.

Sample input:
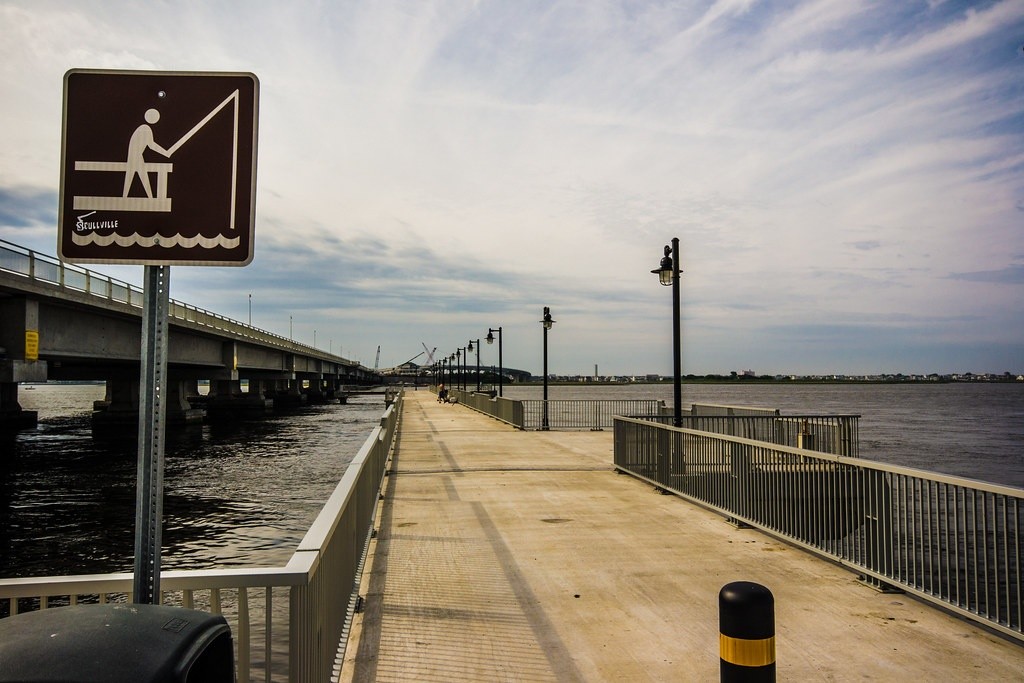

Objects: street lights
[650,245,686,474]
[430,353,456,390]
[248,294,252,325]
[484,327,502,397]
[455,348,466,391]
[539,313,557,431]
[466,339,480,391]
[290,316,293,339]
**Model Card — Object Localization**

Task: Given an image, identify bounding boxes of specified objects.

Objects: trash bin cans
[0,602,236,683]
[799,433,814,463]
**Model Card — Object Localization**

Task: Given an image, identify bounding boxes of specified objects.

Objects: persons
[438,383,446,403]
